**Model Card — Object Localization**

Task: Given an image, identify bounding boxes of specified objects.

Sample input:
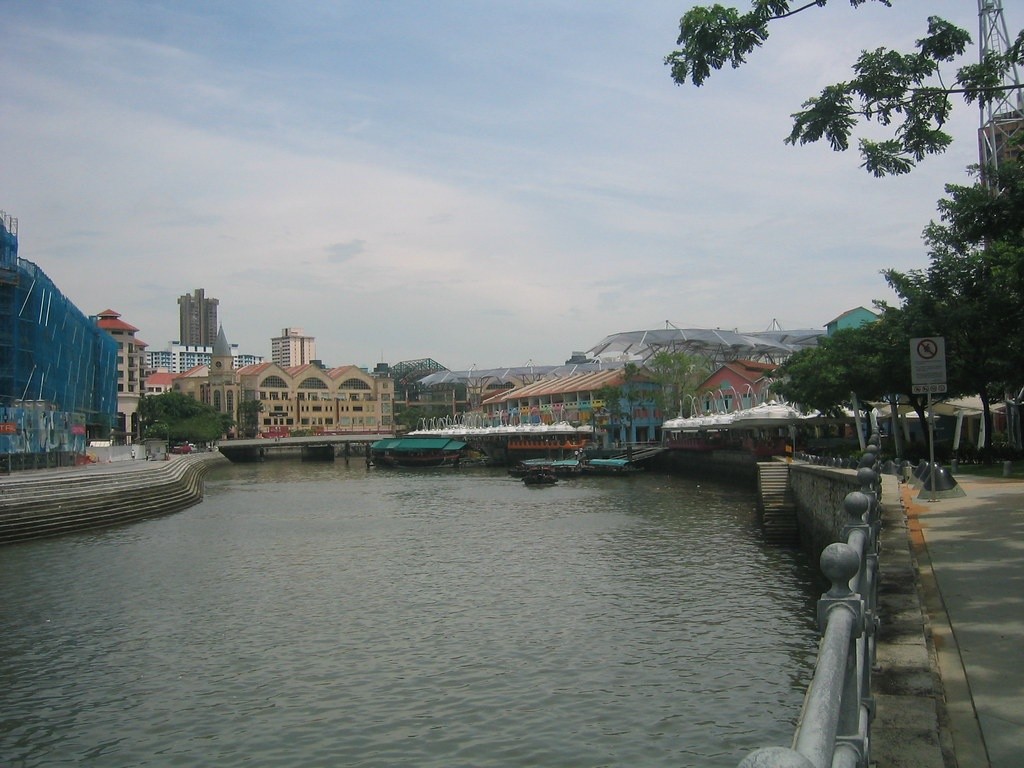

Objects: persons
[131,449,136,461]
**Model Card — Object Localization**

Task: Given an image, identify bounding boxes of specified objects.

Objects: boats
[507,459,628,486]
[371,437,468,468]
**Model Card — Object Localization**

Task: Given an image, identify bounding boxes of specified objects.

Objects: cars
[170,443,197,454]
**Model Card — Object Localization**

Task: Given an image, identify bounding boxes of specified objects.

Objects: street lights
[155,420,170,460]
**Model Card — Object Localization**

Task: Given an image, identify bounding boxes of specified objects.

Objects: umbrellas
[661,399,807,444]
[408,421,599,439]
[806,394,1006,452]
